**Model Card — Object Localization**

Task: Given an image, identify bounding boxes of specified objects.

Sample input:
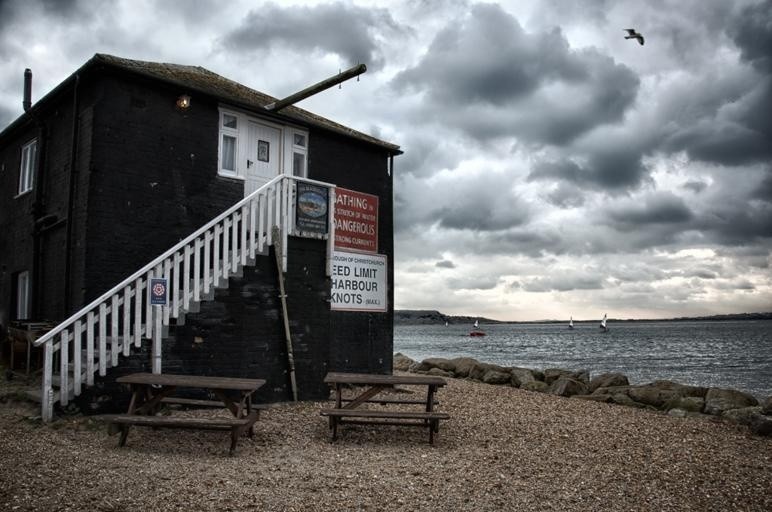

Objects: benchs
[320,408,450,419]
[329,394,439,406]
[145,397,269,410]
[104,413,250,429]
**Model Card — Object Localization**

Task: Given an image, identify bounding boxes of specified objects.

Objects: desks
[115,372,267,456]
[323,371,447,444]
[8,326,59,386]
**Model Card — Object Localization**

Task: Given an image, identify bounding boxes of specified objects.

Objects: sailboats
[569,317,574,328]
[600,313,607,328]
[470,320,486,336]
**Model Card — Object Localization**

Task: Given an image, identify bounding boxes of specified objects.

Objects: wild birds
[625,29,644,46]
